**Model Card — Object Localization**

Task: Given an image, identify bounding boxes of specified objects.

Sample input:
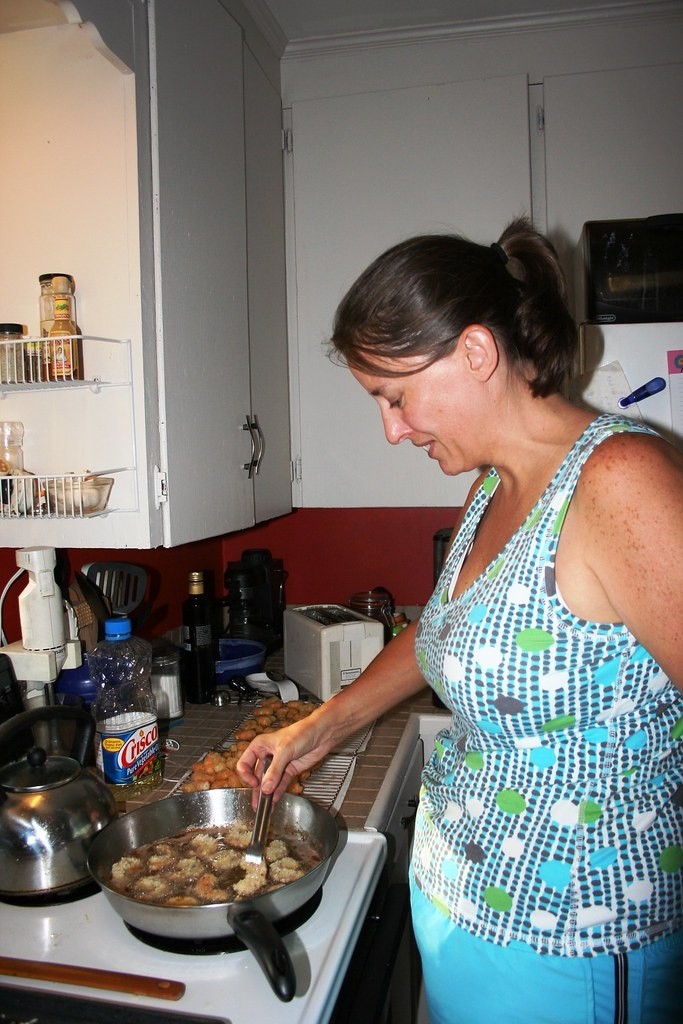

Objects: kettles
[0,704,120,908]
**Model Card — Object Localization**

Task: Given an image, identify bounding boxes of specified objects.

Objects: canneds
[23,273,75,382]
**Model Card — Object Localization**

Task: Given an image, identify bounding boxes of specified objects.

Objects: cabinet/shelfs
[0,336,140,523]
[365,713,452,880]
[275,0,683,513]
[0,0,289,553]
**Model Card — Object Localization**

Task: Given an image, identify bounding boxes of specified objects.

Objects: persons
[236,212,683,1024]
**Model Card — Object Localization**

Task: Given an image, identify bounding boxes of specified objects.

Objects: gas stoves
[1,830,389,1023]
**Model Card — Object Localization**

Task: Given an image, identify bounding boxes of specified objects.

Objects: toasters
[283,602,384,703]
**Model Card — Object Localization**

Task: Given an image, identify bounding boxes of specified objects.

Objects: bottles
[87,618,163,803]
[48,275,80,382]
[0,421,24,474]
[39,273,78,384]
[350,590,393,645]
[181,570,219,706]
[0,323,25,384]
[149,650,184,721]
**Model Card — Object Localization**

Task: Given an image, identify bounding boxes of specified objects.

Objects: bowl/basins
[42,477,114,515]
[216,637,268,686]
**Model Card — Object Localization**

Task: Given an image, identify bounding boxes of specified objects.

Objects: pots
[87,786,340,1002]
